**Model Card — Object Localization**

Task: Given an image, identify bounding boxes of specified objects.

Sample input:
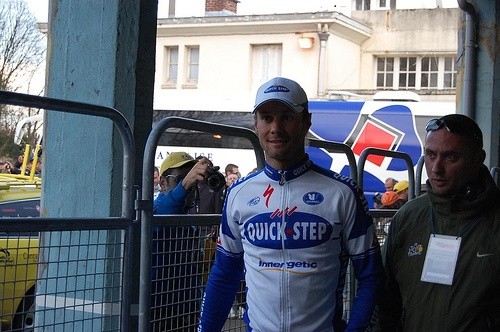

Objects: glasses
[425,119,481,149]
[165,174,187,183]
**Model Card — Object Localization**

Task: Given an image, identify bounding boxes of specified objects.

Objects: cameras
[200,165,227,193]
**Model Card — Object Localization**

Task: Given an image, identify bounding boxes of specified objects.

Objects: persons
[123,150,247,332]
[363,114,500,332]
[371,178,430,232]
[196,77,384,332]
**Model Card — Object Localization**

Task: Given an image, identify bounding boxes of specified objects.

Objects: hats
[393,181,409,194]
[381,191,404,207]
[158,152,199,177]
[251,77,308,114]
